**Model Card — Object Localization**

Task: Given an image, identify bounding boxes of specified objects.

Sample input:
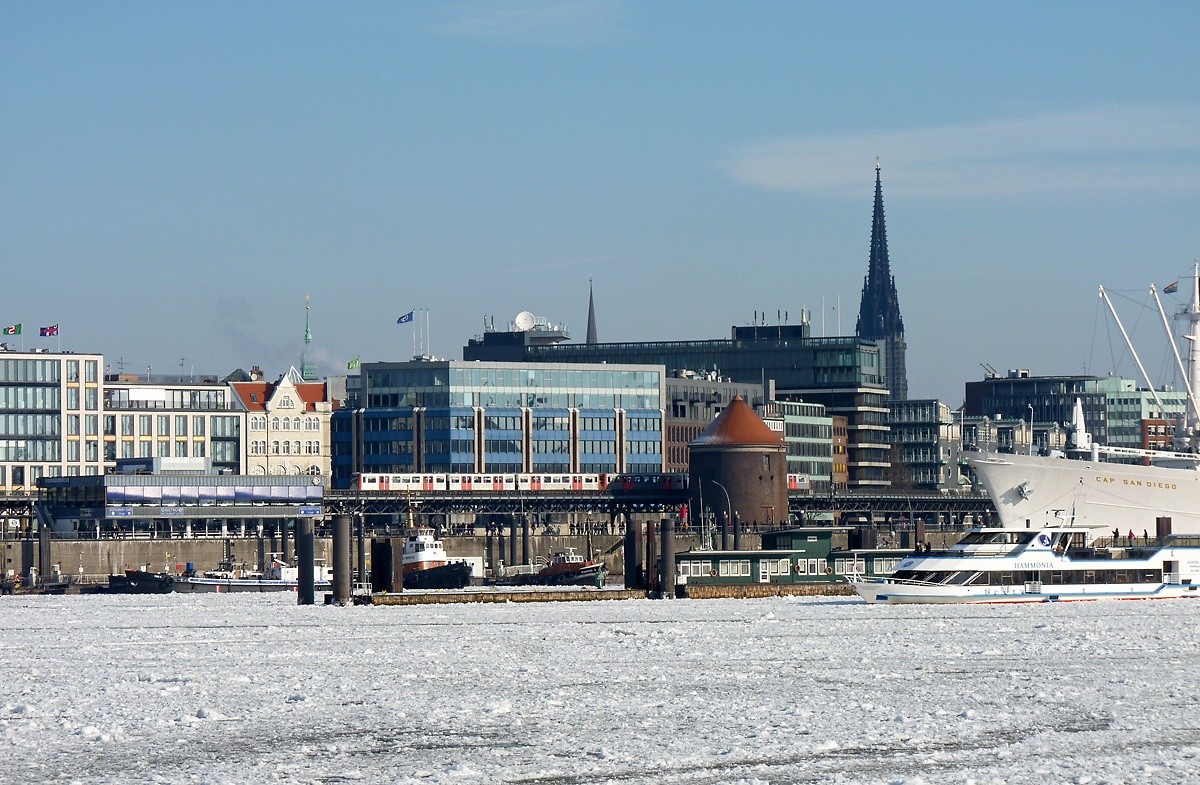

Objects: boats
[105,552,333,600]
[495,528,611,590]
[398,484,475,590]
[851,262,1200,546]
[842,502,1200,599]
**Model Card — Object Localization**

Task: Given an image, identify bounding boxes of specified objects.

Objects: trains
[348,468,812,491]
[602,472,690,490]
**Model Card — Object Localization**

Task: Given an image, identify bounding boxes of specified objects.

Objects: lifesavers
[535,577,545,585]
[165,576,176,587]
[794,565,801,572]
[711,569,717,577]
[545,575,553,584]
[826,567,832,575]
[557,575,567,583]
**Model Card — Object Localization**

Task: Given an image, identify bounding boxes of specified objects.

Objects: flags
[40,323,58,336]
[347,358,360,370]
[3,323,21,335]
[1164,281,1179,293]
[397,311,413,324]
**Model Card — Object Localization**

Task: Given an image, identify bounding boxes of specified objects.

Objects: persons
[25,527,31,541]
[14,525,21,540]
[679,521,690,534]
[954,517,960,532]
[485,522,505,537]
[740,519,790,533]
[531,518,627,536]
[273,561,279,578]
[109,523,129,540]
[1055,542,1065,556]
[176,523,199,539]
[232,524,240,538]
[915,540,932,552]
[1112,528,1148,547]
[978,517,985,525]
[710,528,717,550]
[268,522,276,539]
[78,524,101,539]
[370,523,475,536]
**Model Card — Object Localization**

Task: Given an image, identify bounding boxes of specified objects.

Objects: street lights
[31,499,35,538]
[711,480,731,527]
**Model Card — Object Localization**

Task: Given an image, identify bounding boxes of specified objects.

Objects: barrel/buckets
[186,562,193,571]
